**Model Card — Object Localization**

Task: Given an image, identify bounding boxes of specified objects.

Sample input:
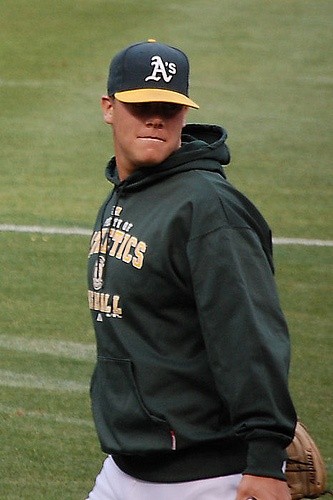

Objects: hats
[107,39,200,110]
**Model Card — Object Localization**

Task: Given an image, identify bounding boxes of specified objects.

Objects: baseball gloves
[285,421,333,500]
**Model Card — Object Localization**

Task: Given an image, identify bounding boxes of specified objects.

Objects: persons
[86,40,297,500]
